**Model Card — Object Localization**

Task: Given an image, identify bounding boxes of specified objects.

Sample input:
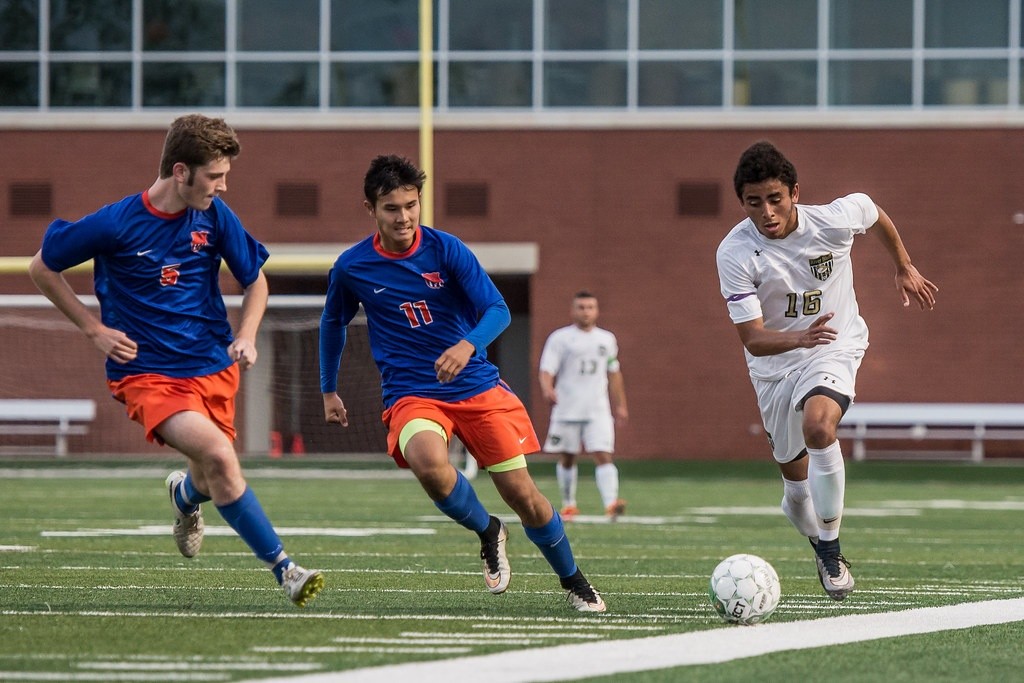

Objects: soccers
[709,552,782,625]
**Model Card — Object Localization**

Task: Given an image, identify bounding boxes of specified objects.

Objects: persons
[320,153,605,612]
[538,290,628,515]
[716,140,938,600]
[29,114,324,609]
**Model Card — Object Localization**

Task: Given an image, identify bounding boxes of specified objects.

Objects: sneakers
[480,515,512,595]
[815,552,855,601]
[280,565,326,608]
[566,581,606,613]
[165,470,205,558]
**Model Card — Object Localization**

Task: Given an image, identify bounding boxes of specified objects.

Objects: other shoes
[607,499,627,517]
[561,506,579,521]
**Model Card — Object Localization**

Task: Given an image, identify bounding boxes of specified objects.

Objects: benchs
[835,403,1023,464]
[0,400,98,458]
